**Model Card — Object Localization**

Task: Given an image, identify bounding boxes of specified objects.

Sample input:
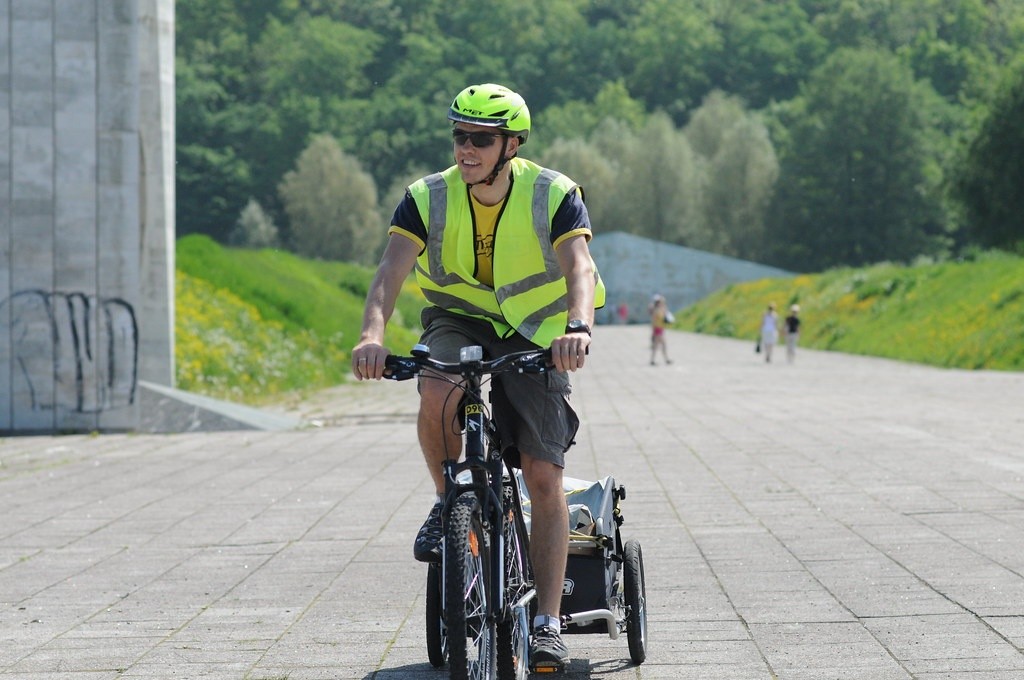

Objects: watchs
[565,318,591,336]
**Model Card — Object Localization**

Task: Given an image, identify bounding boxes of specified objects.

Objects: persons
[784,304,801,359]
[349,84,606,666]
[648,294,674,365]
[757,304,779,363]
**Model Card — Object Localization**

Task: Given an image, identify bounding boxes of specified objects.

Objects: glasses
[451,128,505,147]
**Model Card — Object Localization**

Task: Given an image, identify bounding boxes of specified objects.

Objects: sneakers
[414,493,445,564]
[528,614,571,667]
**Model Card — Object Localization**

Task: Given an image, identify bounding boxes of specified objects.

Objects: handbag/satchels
[756,339,760,352]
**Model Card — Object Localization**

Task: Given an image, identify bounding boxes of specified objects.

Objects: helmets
[447,83,531,146]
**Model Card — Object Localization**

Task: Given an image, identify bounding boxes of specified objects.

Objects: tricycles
[381,343,649,680]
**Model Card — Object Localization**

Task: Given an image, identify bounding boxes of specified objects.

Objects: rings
[359,358,366,361]
